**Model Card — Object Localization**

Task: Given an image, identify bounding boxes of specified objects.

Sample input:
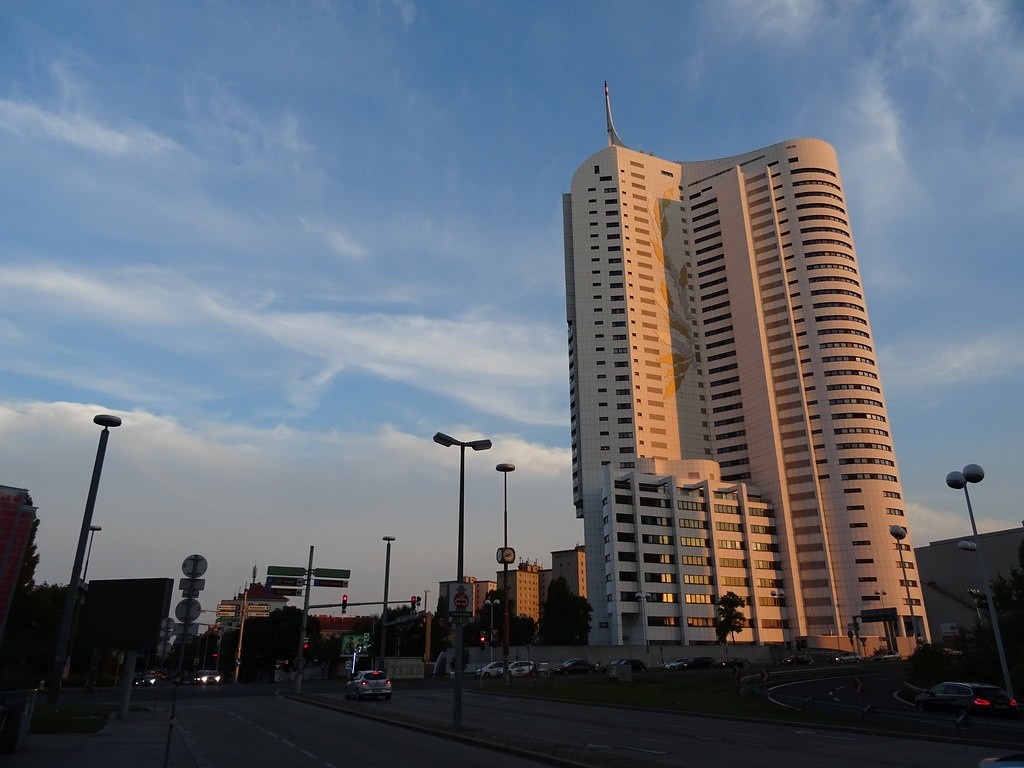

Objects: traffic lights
[304,643,309,648]
[411,596,416,610]
[480,635,486,651]
[342,595,347,610]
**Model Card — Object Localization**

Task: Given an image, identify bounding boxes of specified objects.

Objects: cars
[658,656,751,672]
[549,657,647,675]
[474,660,515,679]
[874,650,902,663]
[497,661,537,678]
[782,654,814,665]
[141,670,222,687]
[833,652,862,664]
[915,681,1018,719]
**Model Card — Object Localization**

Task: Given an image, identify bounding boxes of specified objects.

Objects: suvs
[345,669,392,701]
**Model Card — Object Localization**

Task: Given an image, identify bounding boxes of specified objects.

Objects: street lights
[771,591,787,656]
[890,525,920,649]
[947,465,1013,700]
[378,538,395,672]
[496,464,515,685]
[432,431,493,732]
[635,592,652,668]
[484,599,500,663]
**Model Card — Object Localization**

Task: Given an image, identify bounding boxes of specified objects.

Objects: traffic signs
[267,566,306,576]
[265,588,301,596]
[314,579,348,587]
[266,577,304,587]
[313,569,350,579]
